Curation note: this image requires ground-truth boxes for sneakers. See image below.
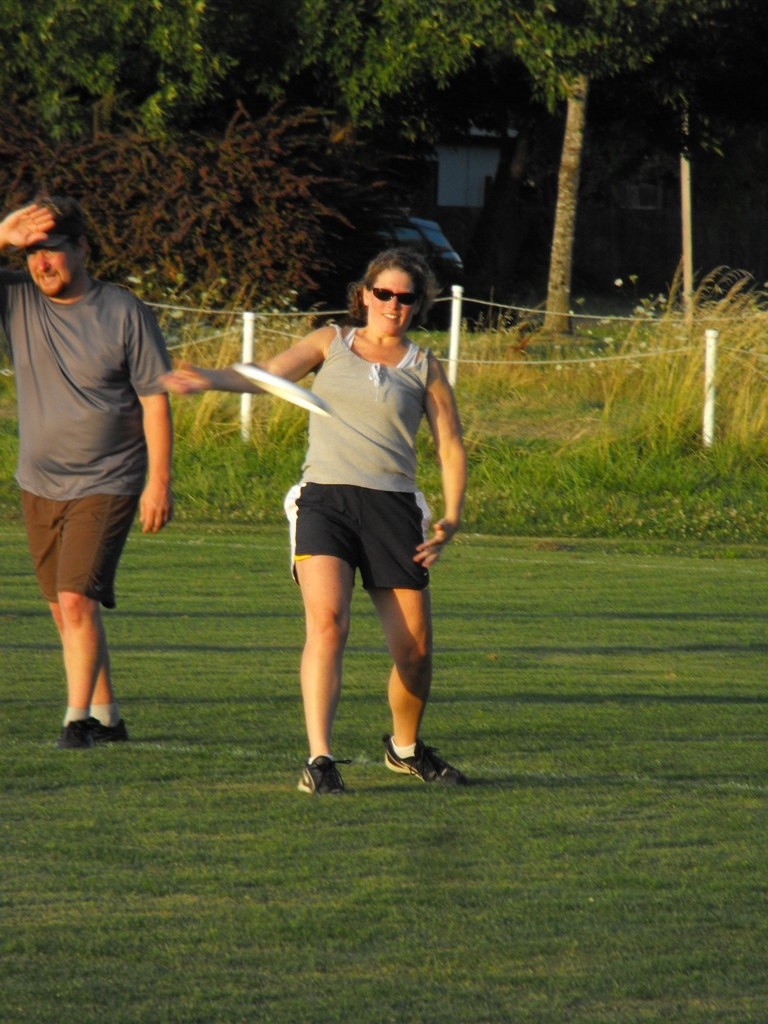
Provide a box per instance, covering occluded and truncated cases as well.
[381,733,467,786]
[85,714,129,745]
[58,717,89,749]
[295,755,352,795]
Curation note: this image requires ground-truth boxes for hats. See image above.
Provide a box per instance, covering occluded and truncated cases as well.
[11,232,68,252]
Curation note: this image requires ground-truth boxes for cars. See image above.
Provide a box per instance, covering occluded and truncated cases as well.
[308,212,464,330]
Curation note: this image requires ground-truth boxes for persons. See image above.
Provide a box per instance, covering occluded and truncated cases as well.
[1,197,176,747]
[156,246,468,790]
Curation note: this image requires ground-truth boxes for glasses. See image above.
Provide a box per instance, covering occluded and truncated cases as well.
[370,285,417,306]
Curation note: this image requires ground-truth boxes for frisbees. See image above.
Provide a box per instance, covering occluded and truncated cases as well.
[231,363,332,420]
